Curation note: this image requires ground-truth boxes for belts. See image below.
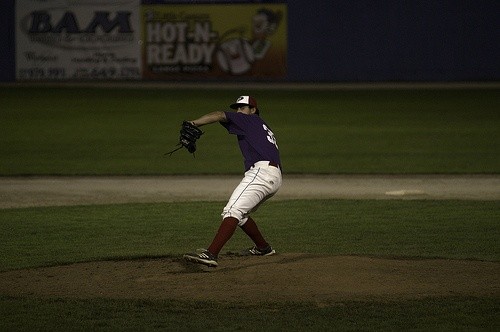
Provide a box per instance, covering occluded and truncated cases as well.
[253,161,279,168]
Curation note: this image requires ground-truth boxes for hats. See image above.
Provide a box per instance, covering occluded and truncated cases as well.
[229,95,257,109]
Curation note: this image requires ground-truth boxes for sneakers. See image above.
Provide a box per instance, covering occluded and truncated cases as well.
[183,248,218,268]
[242,244,275,255]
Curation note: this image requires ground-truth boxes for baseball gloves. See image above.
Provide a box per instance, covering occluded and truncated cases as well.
[178,120,204,155]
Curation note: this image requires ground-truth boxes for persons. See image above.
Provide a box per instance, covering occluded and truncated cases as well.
[181,96,283,266]
